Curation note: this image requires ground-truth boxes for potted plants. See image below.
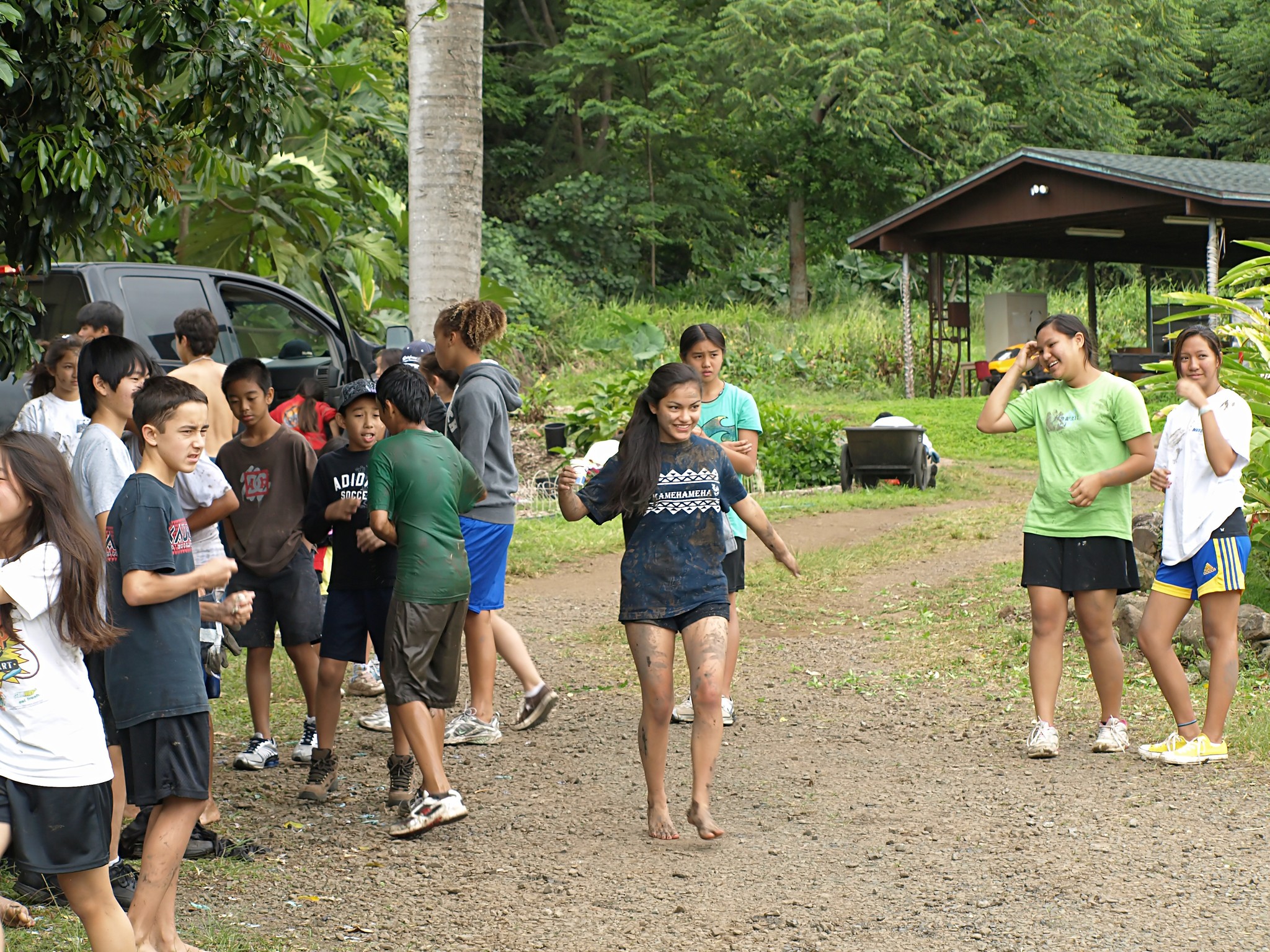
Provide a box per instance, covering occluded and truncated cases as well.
[534,472,558,500]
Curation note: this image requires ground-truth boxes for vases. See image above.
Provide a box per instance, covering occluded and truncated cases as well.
[545,423,567,455]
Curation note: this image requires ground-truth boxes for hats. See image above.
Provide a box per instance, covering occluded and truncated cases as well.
[278,339,315,359]
[337,379,377,412]
[400,340,435,369]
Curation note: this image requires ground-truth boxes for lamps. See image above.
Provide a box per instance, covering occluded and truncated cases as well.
[1030,184,1049,196]
[1162,215,1223,225]
[1065,227,1125,238]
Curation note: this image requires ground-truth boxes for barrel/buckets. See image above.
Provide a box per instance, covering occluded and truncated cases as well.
[535,478,559,499]
[544,423,566,455]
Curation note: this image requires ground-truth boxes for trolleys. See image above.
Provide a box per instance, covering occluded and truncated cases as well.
[840,425,929,491]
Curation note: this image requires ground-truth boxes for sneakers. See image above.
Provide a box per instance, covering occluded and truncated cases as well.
[109,857,140,912]
[1137,732,1189,760]
[349,654,385,697]
[671,687,694,721]
[134,834,213,859]
[233,732,279,769]
[1092,714,1130,753]
[1159,733,1228,764]
[358,705,392,733]
[1027,715,1059,757]
[443,698,502,744]
[389,790,468,839]
[291,716,318,762]
[12,869,70,907]
[509,683,560,731]
[398,786,423,817]
[721,695,736,724]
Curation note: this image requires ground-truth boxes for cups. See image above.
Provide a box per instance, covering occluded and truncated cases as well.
[570,459,589,486]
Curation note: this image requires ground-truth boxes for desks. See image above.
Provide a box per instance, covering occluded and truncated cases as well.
[958,362,976,397]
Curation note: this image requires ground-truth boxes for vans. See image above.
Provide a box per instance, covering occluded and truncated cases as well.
[0,259,414,470]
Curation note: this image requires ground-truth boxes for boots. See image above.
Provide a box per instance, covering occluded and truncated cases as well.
[387,754,415,806]
[297,747,338,802]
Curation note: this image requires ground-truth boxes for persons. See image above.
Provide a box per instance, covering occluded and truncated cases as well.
[658,323,763,724]
[872,411,939,489]
[977,315,1156,760]
[0,298,564,952]
[1136,324,1253,762]
[556,361,802,840]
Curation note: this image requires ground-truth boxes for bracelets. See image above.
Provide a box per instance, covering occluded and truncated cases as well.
[1198,404,1212,415]
